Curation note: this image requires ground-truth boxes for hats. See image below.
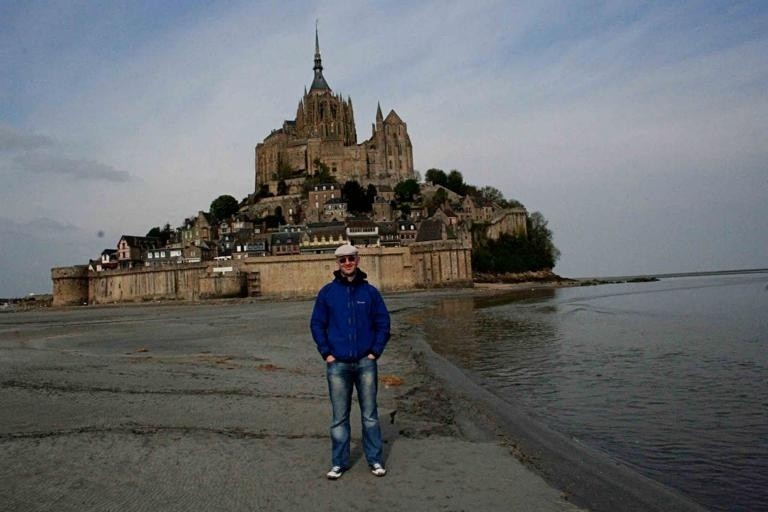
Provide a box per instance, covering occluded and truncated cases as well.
[333,244,359,256]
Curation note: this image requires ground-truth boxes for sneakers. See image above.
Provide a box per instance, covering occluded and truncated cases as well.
[325,465,346,479]
[368,461,387,476]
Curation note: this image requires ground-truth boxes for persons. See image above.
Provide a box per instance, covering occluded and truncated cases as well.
[310,244,390,480]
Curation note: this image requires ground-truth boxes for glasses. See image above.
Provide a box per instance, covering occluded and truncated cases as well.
[336,257,356,263]
